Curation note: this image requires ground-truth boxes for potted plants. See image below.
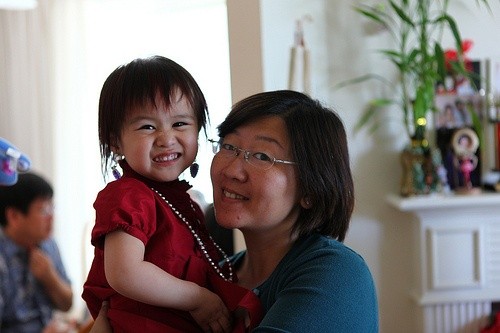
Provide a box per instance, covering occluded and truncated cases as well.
[339,0,495,196]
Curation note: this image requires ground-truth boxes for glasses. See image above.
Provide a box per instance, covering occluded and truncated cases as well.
[208,139,297,168]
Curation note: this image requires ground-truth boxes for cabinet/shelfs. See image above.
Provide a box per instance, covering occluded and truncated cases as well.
[387,191,500,333]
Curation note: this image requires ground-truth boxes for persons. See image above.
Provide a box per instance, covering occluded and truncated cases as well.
[0,171,80,333]
[80,55,225,333]
[82,87,382,333]
[430,66,485,189]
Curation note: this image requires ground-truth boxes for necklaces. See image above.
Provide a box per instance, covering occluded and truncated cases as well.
[144,184,233,285]
